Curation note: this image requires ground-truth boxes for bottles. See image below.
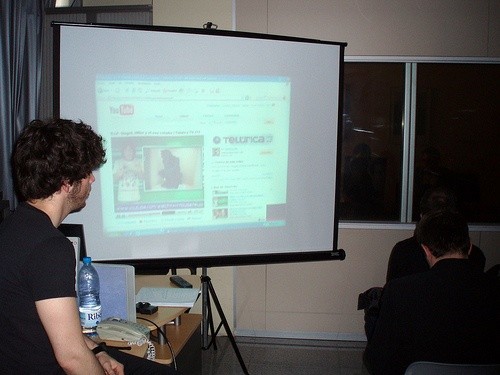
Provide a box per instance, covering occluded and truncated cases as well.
[77,257,102,339]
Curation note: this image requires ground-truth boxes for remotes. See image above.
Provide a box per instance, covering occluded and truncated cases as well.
[170,274,192,290]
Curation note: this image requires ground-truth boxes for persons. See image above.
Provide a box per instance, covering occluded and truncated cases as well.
[0,119,124,375]
[366,190,500,375]
[113,141,145,209]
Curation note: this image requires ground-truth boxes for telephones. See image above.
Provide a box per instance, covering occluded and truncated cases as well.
[96,318,148,342]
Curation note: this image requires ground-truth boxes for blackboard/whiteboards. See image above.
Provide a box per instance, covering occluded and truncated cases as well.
[338,55,500,230]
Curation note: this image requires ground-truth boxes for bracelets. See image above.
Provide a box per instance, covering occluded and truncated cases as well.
[91,346,105,356]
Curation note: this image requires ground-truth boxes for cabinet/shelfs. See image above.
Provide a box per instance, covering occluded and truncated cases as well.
[94,274,203,375]
